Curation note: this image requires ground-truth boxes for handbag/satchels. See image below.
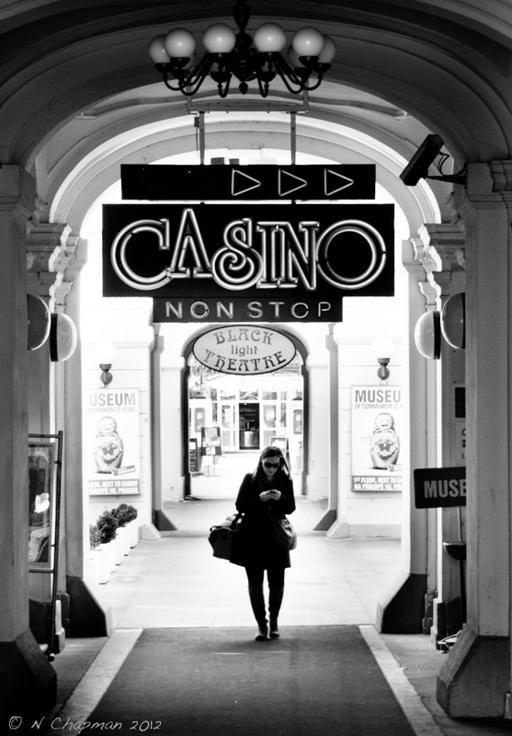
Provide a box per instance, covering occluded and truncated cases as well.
[279,516,298,550]
[207,514,242,560]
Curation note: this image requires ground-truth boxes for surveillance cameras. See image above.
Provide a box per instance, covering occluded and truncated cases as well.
[400,133,444,185]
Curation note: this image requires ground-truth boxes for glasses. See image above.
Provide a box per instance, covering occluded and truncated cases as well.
[264,462,280,468]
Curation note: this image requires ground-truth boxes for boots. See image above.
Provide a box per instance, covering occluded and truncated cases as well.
[254,616,280,642]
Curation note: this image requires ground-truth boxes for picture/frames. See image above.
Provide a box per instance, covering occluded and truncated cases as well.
[81,388,141,496]
[350,384,404,492]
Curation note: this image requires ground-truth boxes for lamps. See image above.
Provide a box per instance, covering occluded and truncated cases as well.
[51,310,78,363]
[100,363,112,386]
[27,293,50,352]
[149,0,336,96]
[414,310,441,360]
[377,358,390,380]
[441,293,466,349]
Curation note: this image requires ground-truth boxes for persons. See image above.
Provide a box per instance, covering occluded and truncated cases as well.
[235,444,298,642]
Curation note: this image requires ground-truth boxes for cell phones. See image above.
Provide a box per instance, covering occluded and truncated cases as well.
[268,490,276,494]
